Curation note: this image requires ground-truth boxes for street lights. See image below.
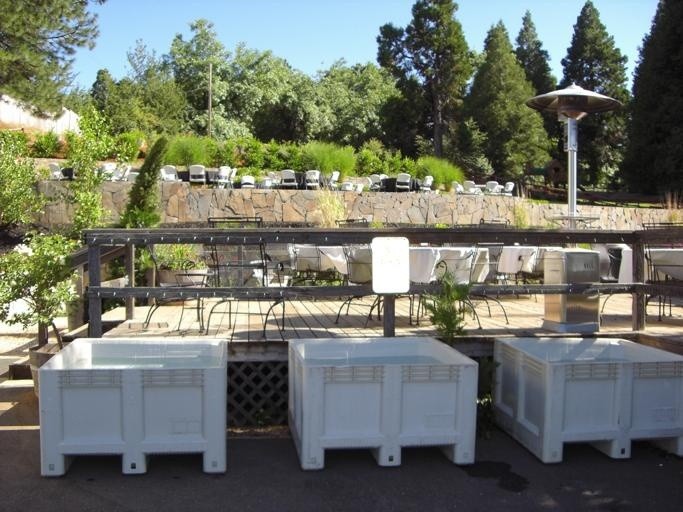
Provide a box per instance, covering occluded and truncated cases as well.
[525,80,622,248]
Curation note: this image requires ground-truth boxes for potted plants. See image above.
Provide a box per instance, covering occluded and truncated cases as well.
[0,231,80,398]
